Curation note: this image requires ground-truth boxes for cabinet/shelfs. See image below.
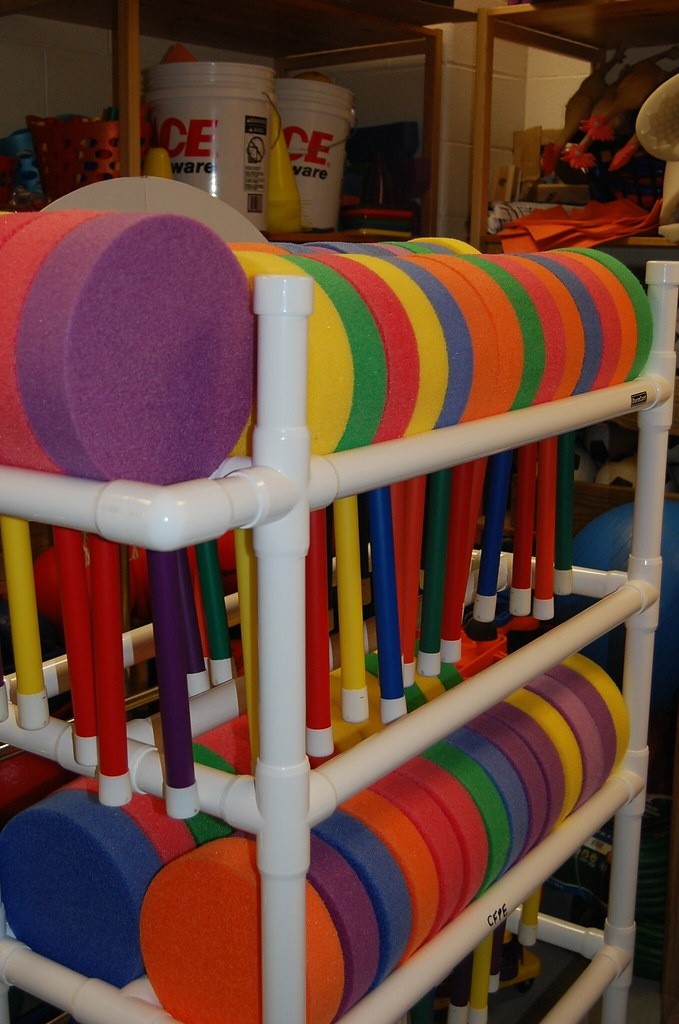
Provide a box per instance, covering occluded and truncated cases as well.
[1,0,679,1024]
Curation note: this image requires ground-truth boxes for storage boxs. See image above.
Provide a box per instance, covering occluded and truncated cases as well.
[513,127,590,205]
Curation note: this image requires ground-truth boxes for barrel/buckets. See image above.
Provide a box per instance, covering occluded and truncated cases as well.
[142,61,278,238]
[272,75,358,233]
